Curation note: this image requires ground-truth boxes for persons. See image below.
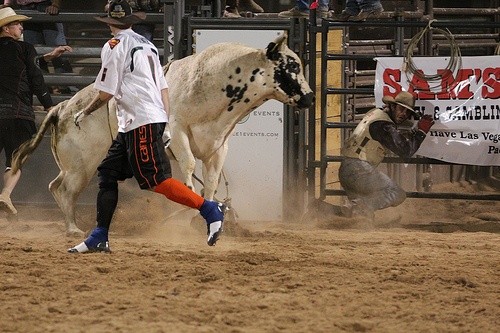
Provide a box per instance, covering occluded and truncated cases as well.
[0,7,56,225]
[277,0,385,21]
[68,0,228,254]
[223,0,265,18]
[306,91,436,227]
[0,0,80,95]
[108,0,165,44]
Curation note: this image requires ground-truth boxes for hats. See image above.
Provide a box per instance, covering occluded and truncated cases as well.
[0,6,32,28]
[96,0,147,26]
[382,91,420,117]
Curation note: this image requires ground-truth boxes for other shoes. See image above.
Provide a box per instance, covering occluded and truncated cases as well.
[349,7,385,22]
[332,13,351,21]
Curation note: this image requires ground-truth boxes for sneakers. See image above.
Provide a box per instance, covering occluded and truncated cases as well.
[278,7,309,20]
[316,10,331,19]
[223,6,241,18]
[67,227,109,253]
[200,200,227,246]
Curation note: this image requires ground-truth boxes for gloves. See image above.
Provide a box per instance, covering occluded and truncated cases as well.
[74,109,88,127]
[162,127,171,148]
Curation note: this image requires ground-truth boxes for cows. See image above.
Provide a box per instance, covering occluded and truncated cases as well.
[7,29,318,239]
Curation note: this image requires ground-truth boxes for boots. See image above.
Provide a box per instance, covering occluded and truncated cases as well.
[0,168,22,217]
